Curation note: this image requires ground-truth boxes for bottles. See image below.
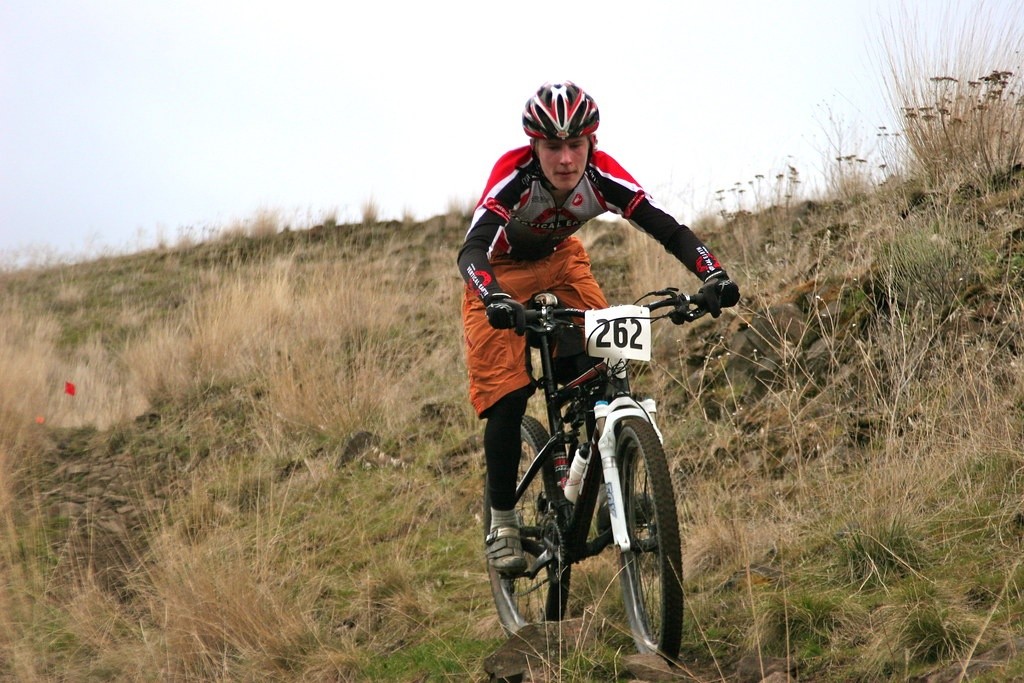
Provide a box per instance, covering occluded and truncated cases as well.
[564,441,591,504]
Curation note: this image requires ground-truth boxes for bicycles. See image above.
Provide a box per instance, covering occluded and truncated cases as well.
[481,287,729,666]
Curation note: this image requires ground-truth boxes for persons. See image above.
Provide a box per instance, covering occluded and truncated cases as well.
[466,80,741,571]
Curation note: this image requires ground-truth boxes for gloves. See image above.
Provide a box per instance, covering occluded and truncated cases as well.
[483,291,526,336]
[697,268,741,318]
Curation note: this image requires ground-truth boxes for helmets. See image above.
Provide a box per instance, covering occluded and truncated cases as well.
[522,81,599,139]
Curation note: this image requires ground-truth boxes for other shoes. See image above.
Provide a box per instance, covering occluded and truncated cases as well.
[486,524,528,575]
[598,498,661,553]
